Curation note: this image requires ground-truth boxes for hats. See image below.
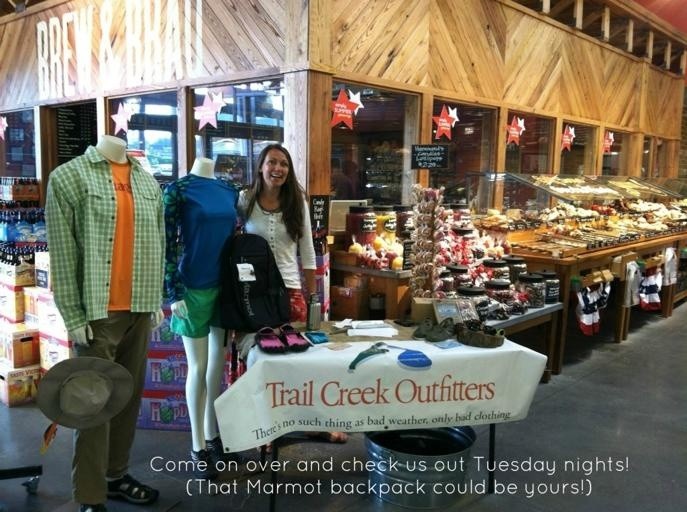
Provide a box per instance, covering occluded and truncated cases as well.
[35,356,135,431]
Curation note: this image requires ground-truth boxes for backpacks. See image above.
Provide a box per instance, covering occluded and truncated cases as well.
[220,233,292,334]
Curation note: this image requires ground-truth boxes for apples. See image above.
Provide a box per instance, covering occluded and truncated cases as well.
[385,252,399,267]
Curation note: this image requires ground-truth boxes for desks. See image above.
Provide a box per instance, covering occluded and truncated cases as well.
[329,256,416,324]
[479,299,563,383]
[206,311,549,511]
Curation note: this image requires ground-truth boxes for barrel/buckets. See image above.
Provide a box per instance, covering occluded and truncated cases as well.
[397,204,411,242]
[346,205,375,247]
[375,205,398,246]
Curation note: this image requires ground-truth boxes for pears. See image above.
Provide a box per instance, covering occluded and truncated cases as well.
[349,243,362,254]
[392,257,403,270]
[372,238,388,251]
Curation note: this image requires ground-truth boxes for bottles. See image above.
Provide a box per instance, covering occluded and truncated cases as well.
[306,293,322,330]
[346,203,560,324]
[0,175,39,208]
[0,207,47,267]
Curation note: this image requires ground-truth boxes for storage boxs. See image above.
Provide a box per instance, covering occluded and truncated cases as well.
[330,273,371,321]
[0,181,328,438]
[406,298,442,323]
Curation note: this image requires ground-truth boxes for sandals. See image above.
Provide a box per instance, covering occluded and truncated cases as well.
[312,431,349,445]
[104,473,159,504]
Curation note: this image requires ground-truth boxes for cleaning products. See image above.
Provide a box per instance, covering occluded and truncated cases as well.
[0,206,46,242]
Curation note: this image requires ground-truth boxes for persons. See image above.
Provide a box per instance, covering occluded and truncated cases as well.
[330,158,357,197]
[162,157,244,481]
[234,145,349,444]
[43,134,166,512]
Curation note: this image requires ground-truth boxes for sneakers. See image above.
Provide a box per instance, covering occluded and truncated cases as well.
[79,503,110,512]
[412,316,456,342]
[204,436,251,467]
[252,325,309,354]
[190,449,217,481]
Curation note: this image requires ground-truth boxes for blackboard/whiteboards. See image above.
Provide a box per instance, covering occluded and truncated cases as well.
[411,144,450,170]
[309,194,329,236]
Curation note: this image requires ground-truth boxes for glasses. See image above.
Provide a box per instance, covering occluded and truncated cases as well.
[468,321,497,336]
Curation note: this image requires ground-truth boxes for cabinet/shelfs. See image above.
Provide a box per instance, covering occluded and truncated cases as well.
[464,168,687,376]
